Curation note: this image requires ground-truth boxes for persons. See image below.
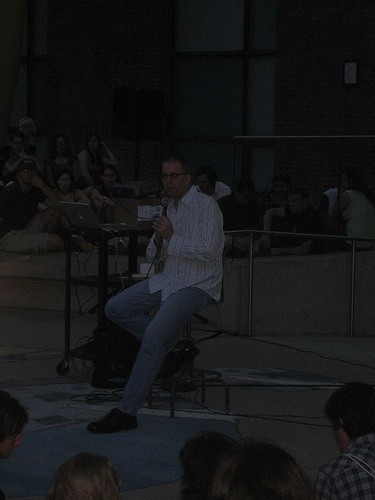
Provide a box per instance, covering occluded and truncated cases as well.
[0,390,29,500]
[46,453,122,500]
[178,431,240,500]
[309,382,375,500]
[0,116,375,259]
[206,443,314,500]
[88,155,225,433]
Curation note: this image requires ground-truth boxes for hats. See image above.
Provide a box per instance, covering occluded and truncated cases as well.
[17,159,39,171]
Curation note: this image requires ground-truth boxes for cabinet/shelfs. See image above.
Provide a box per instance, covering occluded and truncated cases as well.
[41,217,157,376]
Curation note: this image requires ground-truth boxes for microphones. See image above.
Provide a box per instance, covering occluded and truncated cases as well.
[161,198,169,216]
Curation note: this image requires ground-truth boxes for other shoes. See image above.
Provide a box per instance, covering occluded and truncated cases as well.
[85,409,137,432]
[164,342,200,376]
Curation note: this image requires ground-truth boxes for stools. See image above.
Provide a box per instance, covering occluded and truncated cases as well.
[147,275,231,417]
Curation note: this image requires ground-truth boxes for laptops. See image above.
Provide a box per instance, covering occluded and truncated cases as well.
[60,201,134,232]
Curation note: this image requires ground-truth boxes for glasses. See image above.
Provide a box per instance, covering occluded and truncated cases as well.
[161,172,182,178]
